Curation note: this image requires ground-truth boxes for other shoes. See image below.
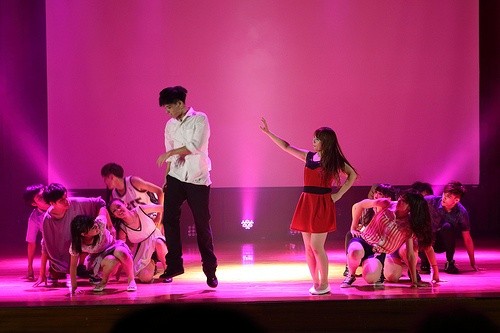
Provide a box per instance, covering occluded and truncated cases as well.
[158,267,184,281]
[93,283,103,291]
[444,259,460,274]
[421,259,430,274]
[339,274,356,287]
[343,266,349,276]
[309,284,331,296]
[374,279,385,289]
[206,271,218,289]
[127,281,137,292]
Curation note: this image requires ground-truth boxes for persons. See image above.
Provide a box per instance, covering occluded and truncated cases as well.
[23,162,169,296]
[259,116,358,295]
[158,85,219,288]
[340,181,486,288]
